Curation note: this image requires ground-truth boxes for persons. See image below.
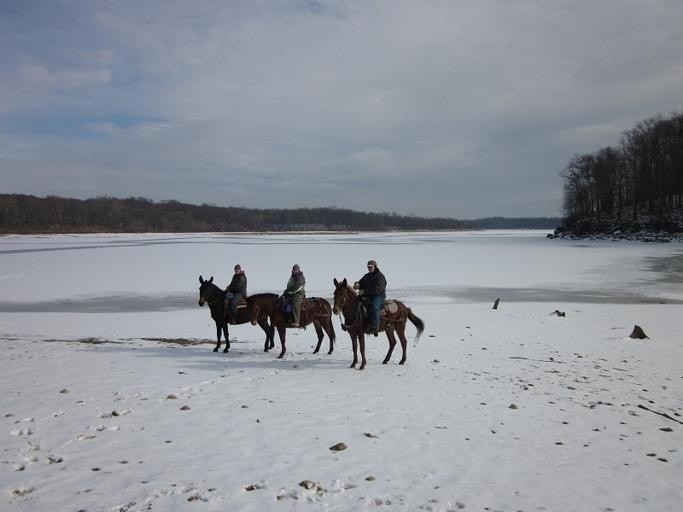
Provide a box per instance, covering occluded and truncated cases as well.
[224,264,247,324]
[283,264,306,327]
[352,259,387,337]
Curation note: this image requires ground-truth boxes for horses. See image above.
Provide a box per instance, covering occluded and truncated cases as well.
[198,275,279,353]
[332,278,424,370]
[246,293,336,358]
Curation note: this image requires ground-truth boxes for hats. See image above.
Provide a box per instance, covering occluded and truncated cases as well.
[234,265,240,271]
[368,261,376,267]
[292,265,299,270]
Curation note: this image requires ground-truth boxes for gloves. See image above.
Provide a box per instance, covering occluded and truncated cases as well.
[359,290,364,295]
[353,283,359,288]
